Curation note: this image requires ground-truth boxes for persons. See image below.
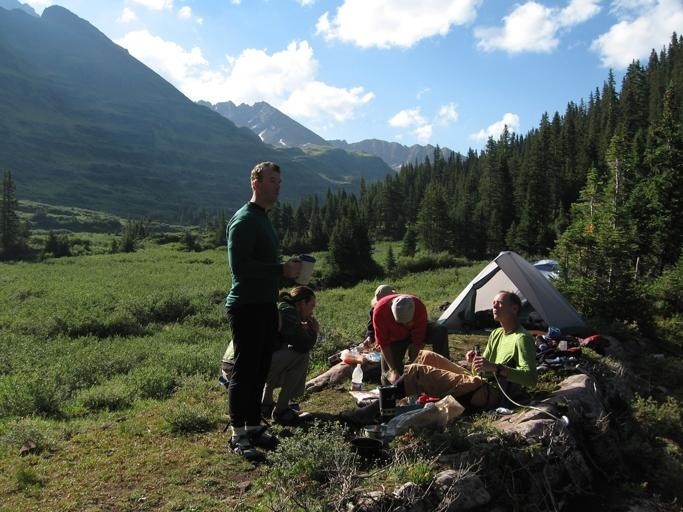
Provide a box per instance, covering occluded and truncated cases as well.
[220,285,321,425]
[341,290,539,425]
[372,294,451,387]
[306,284,398,392]
[225,162,301,463]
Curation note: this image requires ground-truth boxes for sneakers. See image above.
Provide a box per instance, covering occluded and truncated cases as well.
[342,409,377,425]
[360,400,379,411]
[229,402,313,461]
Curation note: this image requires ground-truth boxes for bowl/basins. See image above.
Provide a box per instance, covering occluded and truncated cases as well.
[348,438,384,451]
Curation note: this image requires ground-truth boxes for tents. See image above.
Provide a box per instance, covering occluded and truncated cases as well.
[533,259,576,280]
[437,250,588,335]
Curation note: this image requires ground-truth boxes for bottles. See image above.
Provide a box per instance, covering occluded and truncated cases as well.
[472,343,485,377]
[351,363,364,390]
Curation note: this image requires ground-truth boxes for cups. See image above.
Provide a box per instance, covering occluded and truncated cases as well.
[290,253,317,286]
[378,385,398,420]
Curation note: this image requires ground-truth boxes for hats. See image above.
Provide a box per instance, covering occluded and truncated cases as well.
[371,285,397,308]
[391,295,415,324]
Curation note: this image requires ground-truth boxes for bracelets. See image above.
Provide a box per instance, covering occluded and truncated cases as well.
[495,361,502,376]
